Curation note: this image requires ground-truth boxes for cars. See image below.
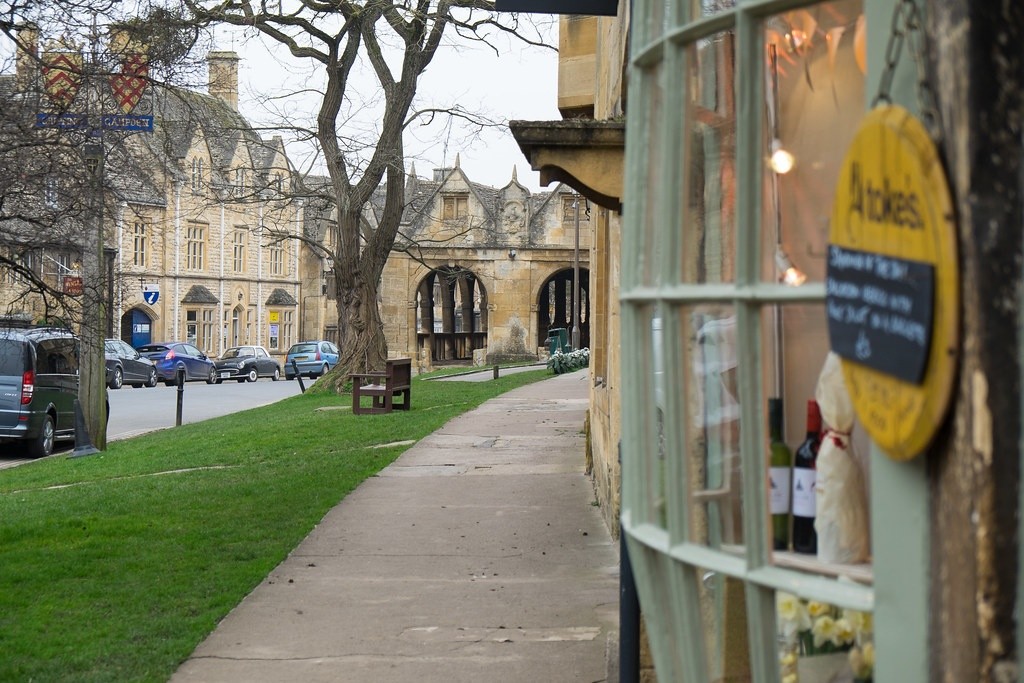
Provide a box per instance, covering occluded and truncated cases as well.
[215,346,281,384]
[104,338,159,390]
[136,342,218,386]
[284,340,339,381]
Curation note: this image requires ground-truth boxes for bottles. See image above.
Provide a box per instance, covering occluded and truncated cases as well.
[792,400,824,555]
[768,398,792,550]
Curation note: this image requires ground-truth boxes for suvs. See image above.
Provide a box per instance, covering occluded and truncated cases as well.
[0,326,111,458]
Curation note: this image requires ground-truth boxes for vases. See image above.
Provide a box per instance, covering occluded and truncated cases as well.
[798,652,845,683]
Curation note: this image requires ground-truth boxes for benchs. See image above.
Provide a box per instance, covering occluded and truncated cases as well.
[349,359,414,415]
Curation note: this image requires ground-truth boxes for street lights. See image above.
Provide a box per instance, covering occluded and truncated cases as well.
[256,190,268,345]
[172,174,183,344]
[219,185,228,354]
[570,188,581,352]
[117,192,129,341]
[294,196,303,340]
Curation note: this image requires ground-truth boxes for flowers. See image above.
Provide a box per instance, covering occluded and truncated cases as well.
[774,590,874,683]
